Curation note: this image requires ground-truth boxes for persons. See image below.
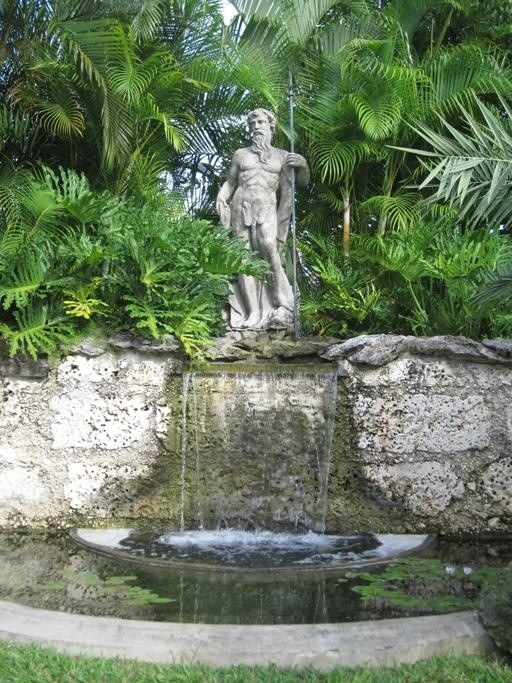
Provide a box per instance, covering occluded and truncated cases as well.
[212,109,315,337]
